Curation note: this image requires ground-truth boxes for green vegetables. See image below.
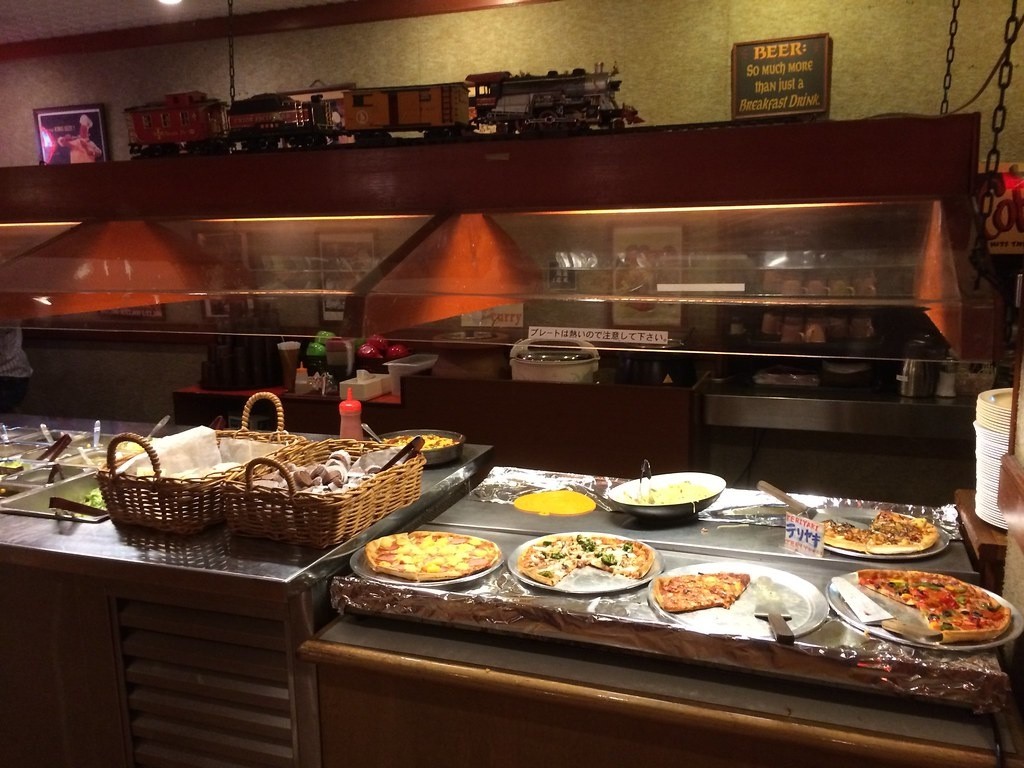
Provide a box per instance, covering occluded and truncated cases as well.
[82,487,107,510]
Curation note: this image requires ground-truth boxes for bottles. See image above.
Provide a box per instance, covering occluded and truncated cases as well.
[295,363,311,395]
[901,339,957,398]
[340,390,363,443]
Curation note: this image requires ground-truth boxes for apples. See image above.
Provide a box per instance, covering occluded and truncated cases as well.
[306,330,338,355]
[354,334,410,359]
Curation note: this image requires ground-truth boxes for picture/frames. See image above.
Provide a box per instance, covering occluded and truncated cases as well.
[32,103,109,165]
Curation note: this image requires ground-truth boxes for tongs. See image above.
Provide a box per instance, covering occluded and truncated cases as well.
[210,415,226,431]
[50,495,107,517]
[38,434,72,460]
[377,437,425,474]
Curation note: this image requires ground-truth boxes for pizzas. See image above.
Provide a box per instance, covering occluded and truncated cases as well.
[380,434,460,450]
[365,530,501,581]
[818,512,939,554]
[855,569,1010,642]
[653,572,751,613]
[517,533,655,586]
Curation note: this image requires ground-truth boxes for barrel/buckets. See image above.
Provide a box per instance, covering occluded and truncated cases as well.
[616,337,695,390]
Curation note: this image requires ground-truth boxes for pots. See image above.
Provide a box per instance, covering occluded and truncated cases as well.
[510,336,600,384]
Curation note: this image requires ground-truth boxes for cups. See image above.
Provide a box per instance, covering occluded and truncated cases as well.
[765,270,878,297]
[762,308,875,342]
[277,341,300,388]
[326,336,355,378]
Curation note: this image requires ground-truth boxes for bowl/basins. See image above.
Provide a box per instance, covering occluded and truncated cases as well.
[374,430,466,465]
[609,472,727,524]
[360,359,396,372]
[302,355,326,372]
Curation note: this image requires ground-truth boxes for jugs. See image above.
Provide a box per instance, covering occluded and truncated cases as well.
[727,316,747,352]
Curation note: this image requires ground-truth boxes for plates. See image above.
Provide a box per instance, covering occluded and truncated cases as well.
[350,546,502,586]
[648,561,831,636]
[827,570,1023,653]
[504,531,665,594]
[972,388,1013,530]
[792,508,951,558]
